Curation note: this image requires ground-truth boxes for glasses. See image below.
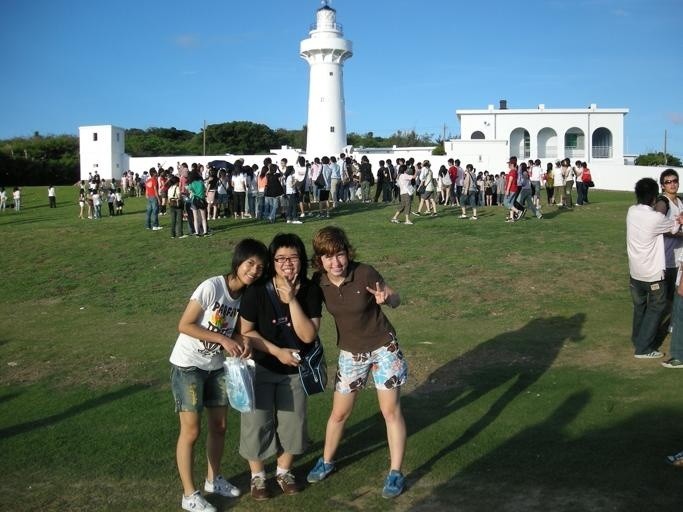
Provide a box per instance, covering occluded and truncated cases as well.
[664,179,679,185]
[274,255,300,263]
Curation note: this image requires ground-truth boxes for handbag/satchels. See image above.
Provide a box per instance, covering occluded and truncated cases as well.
[193,195,208,209]
[416,177,433,195]
[581,177,594,187]
[343,161,350,185]
[169,185,183,209]
[295,166,309,195]
[314,165,326,188]
[219,179,233,201]
[406,184,415,196]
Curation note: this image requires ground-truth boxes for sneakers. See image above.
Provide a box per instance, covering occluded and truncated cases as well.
[536,210,543,220]
[205,475,242,498]
[411,211,421,217]
[515,210,523,220]
[391,217,400,224]
[275,470,300,496]
[634,351,665,359]
[429,206,433,210]
[291,220,303,225]
[179,235,189,239]
[666,451,683,467]
[523,207,527,220]
[263,278,328,396]
[662,358,683,369]
[146,227,151,230]
[250,474,271,503]
[307,213,314,217]
[287,220,292,223]
[469,217,478,221]
[195,234,200,238]
[170,236,176,239]
[202,232,212,237]
[299,214,305,218]
[153,226,163,231]
[505,217,514,222]
[326,211,331,218]
[423,211,432,215]
[307,457,338,483]
[382,470,406,499]
[431,213,438,217]
[457,214,467,219]
[316,214,325,218]
[181,490,217,512]
[404,221,413,225]
[268,216,274,224]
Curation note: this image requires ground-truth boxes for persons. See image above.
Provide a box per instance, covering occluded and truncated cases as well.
[294,156,314,217]
[561,160,567,178]
[345,156,374,204]
[231,167,247,220]
[409,158,415,185]
[168,239,268,512]
[279,158,287,218]
[405,161,413,175]
[107,191,116,216]
[563,158,575,209]
[314,156,332,218]
[80,181,86,191]
[89,172,93,181]
[79,189,85,219]
[92,190,102,219]
[145,168,163,230]
[507,157,526,219]
[92,182,98,193]
[436,177,441,204]
[662,213,683,369]
[572,160,584,206]
[390,166,418,225]
[504,160,522,222]
[181,163,189,176]
[440,165,452,205]
[551,161,563,206]
[413,161,438,217]
[204,170,218,219]
[520,164,543,218]
[13,186,21,211]
[85,192,95,218]
[373,160,386,202]
[186,174,208,235]
[305,160,314,168]
[457,164,477,220]
[236,232,322,500]
[101,179,107,191]
[246,167,257,218]
[264,164,283,223]
[476,171,505,206]
[216,170,230,219]
[308,227,407,500]
[527,160,533,178]
[330,157,341,207]
[455,159,464,207]
[383,159,394,203]
[141,172,147,183]
[394,159,400,202]
[120,170,134,193]
[169,167,173,175]
[424,198,434,214]
[447,159,457,206]
[158,168,164,196]
[192,161,211,178]
[167,177,188,239]
[310,158,321,203]
[264,158,272,167]
[94,171,100,184]
[625,178,683,359]
[133,173,141,197]
[659,170,683,332]
[284,166,304,224]
[48,184,56,208]
[115,188,124,215]
[111,178,117,193]
[397,159,405,173]
[257,166,269,220]
[252,164,258,172]
[0,187,7,211]
[160,170,170,215]
[581,162,594,202]
[655,197,669,336]
[543,163,554,204]
[88,179,93,191]
[532,159,542,209]
[337,153,346,202]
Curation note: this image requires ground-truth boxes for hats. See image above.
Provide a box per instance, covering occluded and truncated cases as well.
[506,159,517,165]
